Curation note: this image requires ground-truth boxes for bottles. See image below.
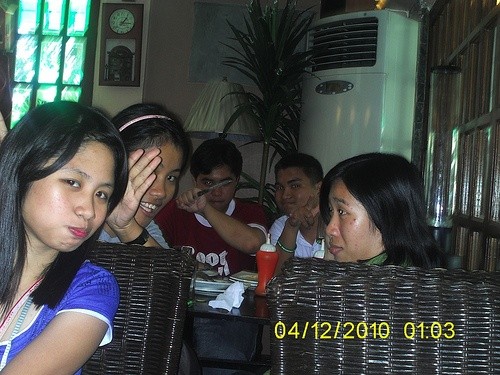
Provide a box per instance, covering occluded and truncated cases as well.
[257,233,279,296]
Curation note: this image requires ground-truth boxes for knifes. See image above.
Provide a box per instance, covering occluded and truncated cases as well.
[193,180,231,198]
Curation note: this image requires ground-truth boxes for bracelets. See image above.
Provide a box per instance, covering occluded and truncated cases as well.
[125,228,149,245]
[277,238,297,253]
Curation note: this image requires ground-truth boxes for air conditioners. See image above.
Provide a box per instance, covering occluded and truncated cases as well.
[293,13,421,176]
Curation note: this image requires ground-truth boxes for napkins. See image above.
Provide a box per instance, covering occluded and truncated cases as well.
[209,280,246,312]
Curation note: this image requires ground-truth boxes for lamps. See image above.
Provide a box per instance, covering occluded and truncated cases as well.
[184,75,263,144]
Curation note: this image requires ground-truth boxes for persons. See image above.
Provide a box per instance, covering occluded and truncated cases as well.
[268,153,324,277]
[0,100,129,375]
[96,102,189,248]
[319,153,441,268]
[152,139,269,280]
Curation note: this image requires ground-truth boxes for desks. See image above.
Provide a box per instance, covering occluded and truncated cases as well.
[183,270,271,375]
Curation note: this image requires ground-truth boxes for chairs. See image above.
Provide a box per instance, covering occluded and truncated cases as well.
[80,243,192,375]
[262,251,500,375]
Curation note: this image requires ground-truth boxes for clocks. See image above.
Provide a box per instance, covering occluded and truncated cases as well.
[97,2,145,89]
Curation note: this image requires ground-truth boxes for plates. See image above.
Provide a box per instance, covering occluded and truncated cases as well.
[190,287,225,297]
[195,278,231,289]
[229,276,258,289]
